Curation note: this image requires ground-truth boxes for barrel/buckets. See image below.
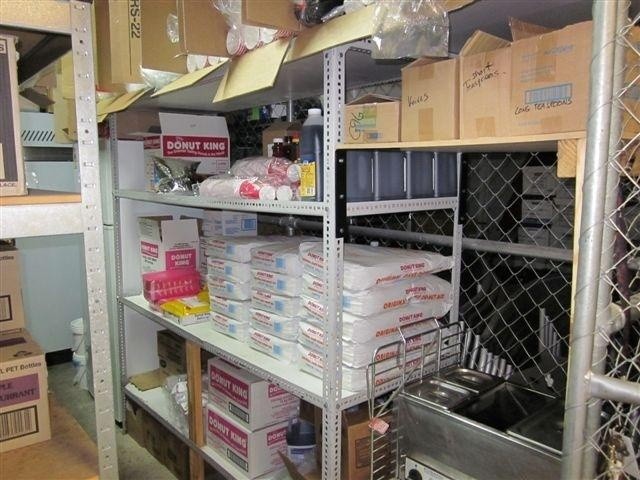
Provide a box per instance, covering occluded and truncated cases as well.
[69,317,86,353]
[72,353,88,390]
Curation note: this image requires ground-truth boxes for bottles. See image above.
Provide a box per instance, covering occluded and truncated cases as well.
[271,133,300,163]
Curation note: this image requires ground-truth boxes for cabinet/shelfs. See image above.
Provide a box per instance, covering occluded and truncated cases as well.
[0,1,640,480]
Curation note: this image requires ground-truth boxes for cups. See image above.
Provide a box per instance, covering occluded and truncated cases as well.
[186,54,230,71]
[198,156,302,202]
[226,22,297,56]
